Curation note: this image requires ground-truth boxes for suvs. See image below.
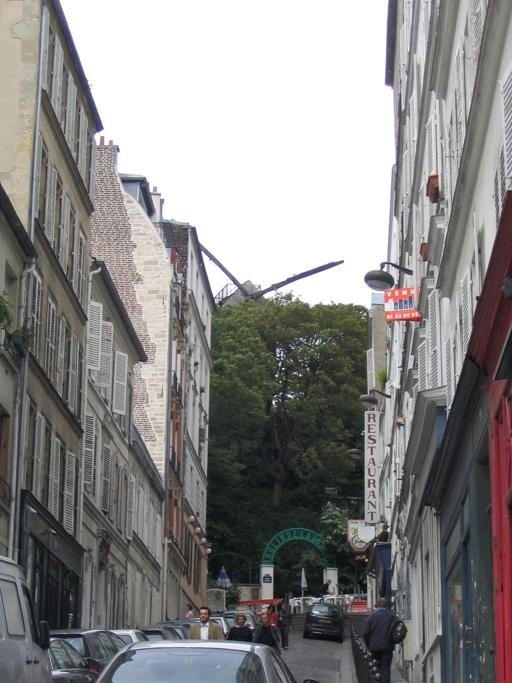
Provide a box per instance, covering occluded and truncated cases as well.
[0,555,55,683]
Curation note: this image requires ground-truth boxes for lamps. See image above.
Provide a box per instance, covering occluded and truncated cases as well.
[363,261,414,292]
[187,514,211,556]
[358,388,391,408]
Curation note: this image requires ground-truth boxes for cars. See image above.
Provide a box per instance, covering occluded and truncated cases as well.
[94,638,319,683]
[47,637,95,683]
[111,629,151,644]
[48,629,128,675]
[132,609,260,643]
[302,599,346,642]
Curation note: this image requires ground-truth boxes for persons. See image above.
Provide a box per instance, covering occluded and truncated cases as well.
[254,610,282,656]
[277,602,293,649]
[184,602,193,618]
[361,597,400,683]
[317,579,331,594]
[187,605,225,640]
[266,603,278,627]
[227,612,253,642]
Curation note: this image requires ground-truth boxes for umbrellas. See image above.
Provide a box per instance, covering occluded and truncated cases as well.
[300,567,308,596]
[213,565,233,609]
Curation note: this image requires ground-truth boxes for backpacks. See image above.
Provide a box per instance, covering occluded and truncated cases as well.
[389,613,407,644]
